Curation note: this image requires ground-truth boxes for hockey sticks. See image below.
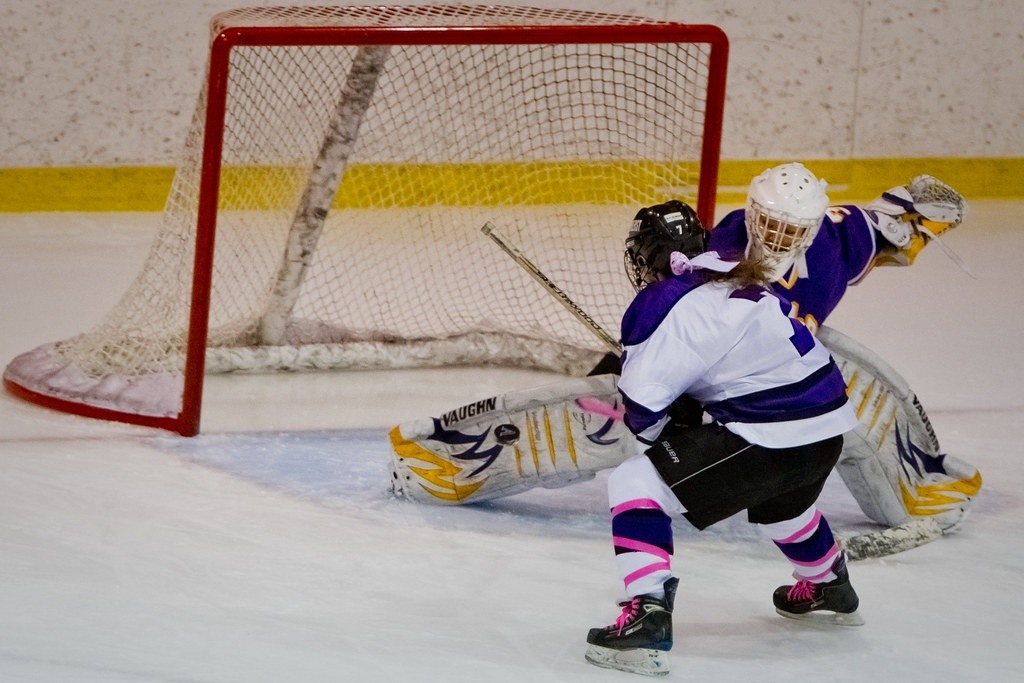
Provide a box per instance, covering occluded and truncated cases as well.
[478,217,946,567]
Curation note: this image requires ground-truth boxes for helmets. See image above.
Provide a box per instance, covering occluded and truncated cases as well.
[625,199,708,276]
[745,162,831,285]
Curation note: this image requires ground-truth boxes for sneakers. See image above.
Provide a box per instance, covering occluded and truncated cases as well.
[584,575,680,677]
[774,547,865,630]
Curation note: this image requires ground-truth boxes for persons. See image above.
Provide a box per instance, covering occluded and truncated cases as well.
[388,157,981,678]
